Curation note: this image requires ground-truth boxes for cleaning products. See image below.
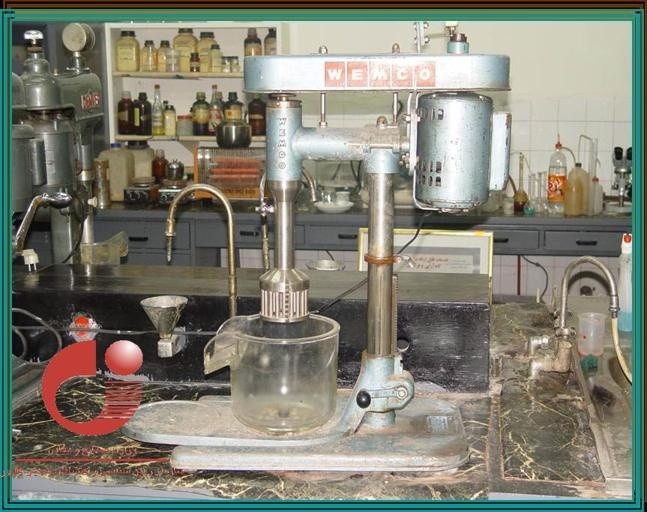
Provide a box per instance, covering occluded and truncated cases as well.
[616,232,633,331]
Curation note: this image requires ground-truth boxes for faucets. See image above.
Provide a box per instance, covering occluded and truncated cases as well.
[163,182,239,318]
[257,166,318,204]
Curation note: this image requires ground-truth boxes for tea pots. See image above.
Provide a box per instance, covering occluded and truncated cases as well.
[211,92,251,147]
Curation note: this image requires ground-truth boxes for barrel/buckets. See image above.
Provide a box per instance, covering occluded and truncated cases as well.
[568,163,589,215]
[123,139,154,180]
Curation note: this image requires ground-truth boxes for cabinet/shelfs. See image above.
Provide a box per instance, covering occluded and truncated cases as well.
[13,209,632,316]
[105,20,290,175]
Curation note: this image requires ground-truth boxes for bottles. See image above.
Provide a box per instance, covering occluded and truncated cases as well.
[186,173,195,201]
[514,139,605,214]
[113,31,278,136]
[618,233,633,332]
[447,34,468,54]
[153,149,166,176]
[127,140,155,189]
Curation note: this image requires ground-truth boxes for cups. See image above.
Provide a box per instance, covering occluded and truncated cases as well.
[227,314,337,433]
[79,242,121,266]
[168,160,183,181]
